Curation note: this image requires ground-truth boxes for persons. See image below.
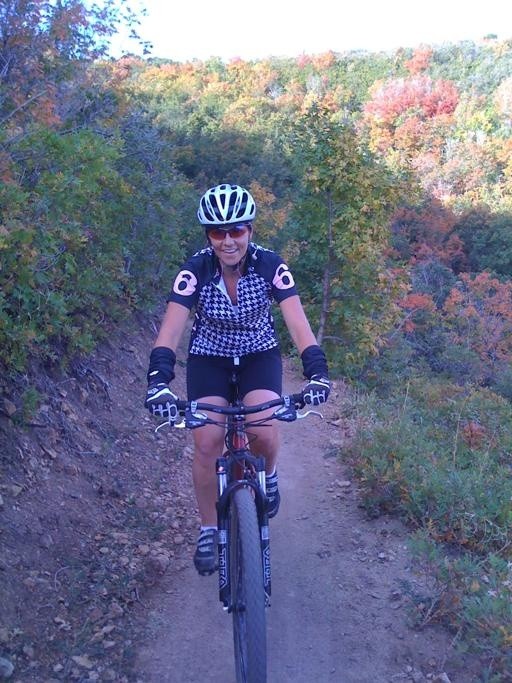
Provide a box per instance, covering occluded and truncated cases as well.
[145,184,331,572]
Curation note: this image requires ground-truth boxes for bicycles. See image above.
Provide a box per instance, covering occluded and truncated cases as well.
[155,390,323,683]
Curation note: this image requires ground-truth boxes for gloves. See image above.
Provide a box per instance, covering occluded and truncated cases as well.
[144,382,180,427]
[302,374,331,406]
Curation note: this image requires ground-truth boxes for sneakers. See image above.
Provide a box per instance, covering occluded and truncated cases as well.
[265,470,281,518]
[193,528,218,572]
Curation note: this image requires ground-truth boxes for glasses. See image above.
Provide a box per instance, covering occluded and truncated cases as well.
[207,227,249,240]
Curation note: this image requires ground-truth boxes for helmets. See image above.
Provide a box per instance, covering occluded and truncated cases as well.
[196,183,257,226]
